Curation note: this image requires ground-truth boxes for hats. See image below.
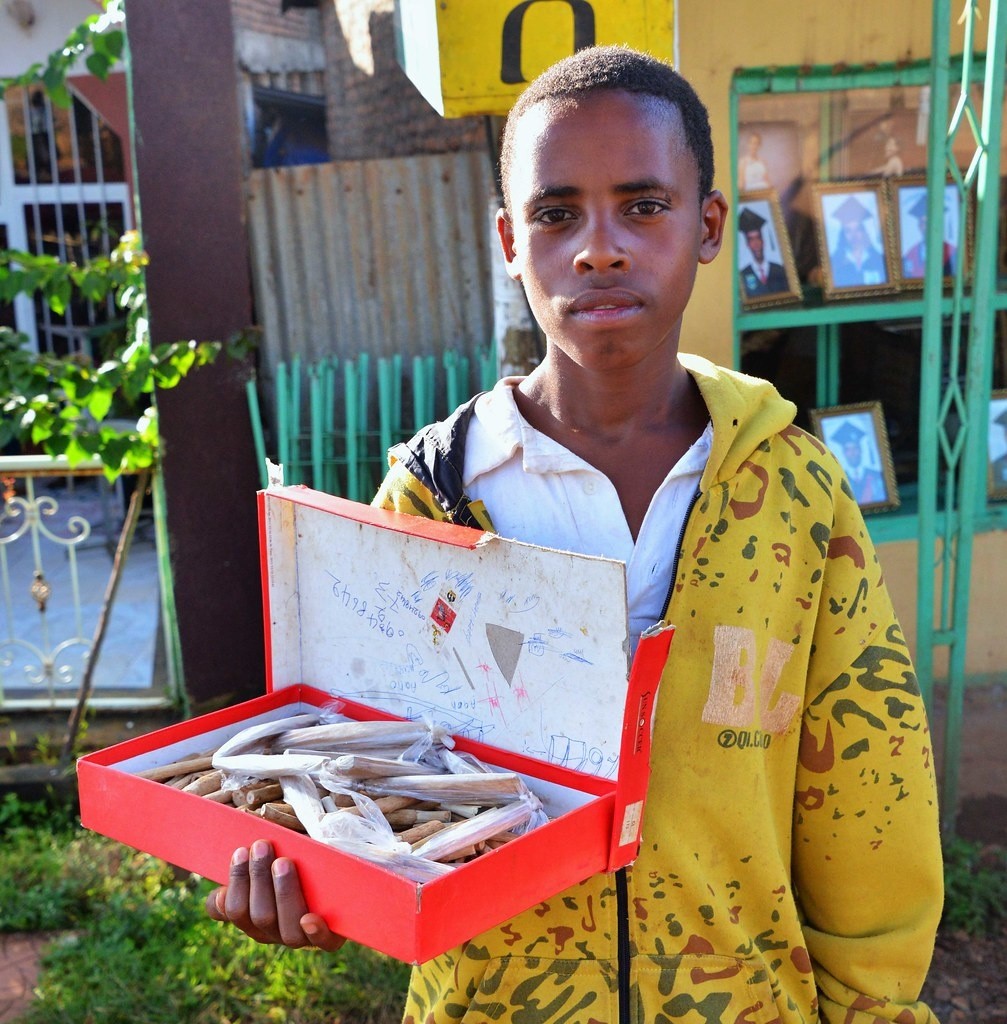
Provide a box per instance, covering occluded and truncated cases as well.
[907,193,953,240]
[832,420,875,465]
[739,209,776,252]
[832,196,880,242]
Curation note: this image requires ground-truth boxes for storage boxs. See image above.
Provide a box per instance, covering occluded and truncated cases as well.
[74,482,678,966]
[812,400,903,517]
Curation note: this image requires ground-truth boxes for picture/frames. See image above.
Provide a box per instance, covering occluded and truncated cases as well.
[807,179,900,300]
[738,189,805,312]
[890,173,973,290]
[965,390,1007,501]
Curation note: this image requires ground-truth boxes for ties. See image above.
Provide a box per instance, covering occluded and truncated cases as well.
[758,265,767,284]
[854,467,861,481]
[852,244,862,267]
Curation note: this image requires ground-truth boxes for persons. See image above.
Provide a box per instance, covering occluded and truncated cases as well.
[207,47,944,1024]
[902,195,957,279]
[739,208,790,297]
[832,423,888,505]
[866,136,904,178]
[738,133,771,191]
[830,198,885,287]
[993,409,1007,486]
